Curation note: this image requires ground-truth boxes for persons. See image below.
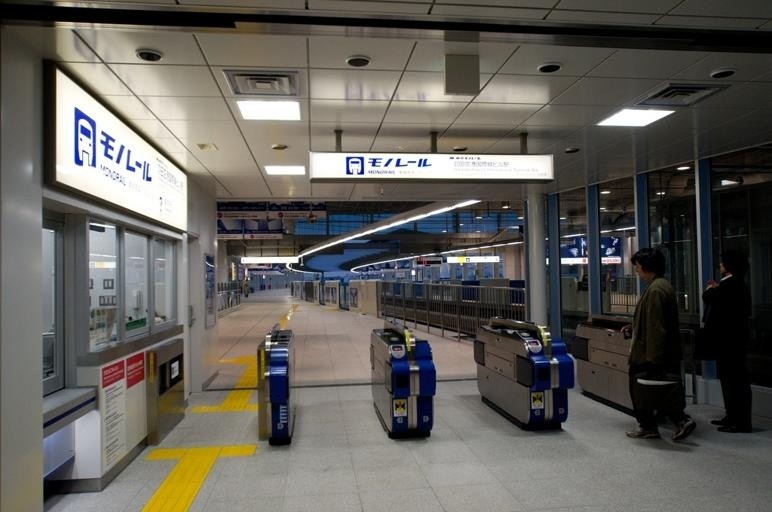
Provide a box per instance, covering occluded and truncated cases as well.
[699,252,755,433]
[621,247,697,443]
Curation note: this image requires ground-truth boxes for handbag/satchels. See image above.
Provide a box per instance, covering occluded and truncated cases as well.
[634,368,682,415]
[694,329,716,361]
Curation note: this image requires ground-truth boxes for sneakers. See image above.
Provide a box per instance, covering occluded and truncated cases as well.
[626,428,660,440]
[671,417,696,440]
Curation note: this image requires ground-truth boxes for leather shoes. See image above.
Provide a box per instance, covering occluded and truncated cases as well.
[712,417,753,432]
